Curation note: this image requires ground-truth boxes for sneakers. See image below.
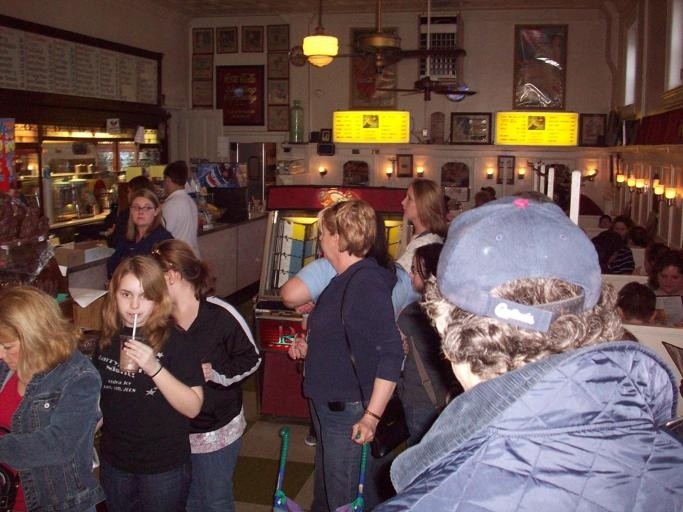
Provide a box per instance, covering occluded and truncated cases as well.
[305,432,318,446]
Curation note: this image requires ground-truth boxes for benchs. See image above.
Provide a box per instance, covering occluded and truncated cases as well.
[576,214,682,421]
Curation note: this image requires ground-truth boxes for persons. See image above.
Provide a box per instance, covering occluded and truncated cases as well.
[107,160,201,281]
[0,285,106,512]
[370,195,683,512]
[475,187,496,207]
[280,181,465,512]
[591,215,683,371]
[154,241,265,512]
[94,255,207,512]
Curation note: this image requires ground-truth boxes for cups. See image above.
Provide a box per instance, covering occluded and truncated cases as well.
[119,335,144,374]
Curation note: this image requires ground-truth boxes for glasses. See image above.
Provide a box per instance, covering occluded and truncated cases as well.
[128,206,155,211]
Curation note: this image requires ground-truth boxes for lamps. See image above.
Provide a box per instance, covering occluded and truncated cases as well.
[517,167,527,179]
[386,167,393,178]
[302,0,338,68]
[318,166,327,176]
[486,168,494,179]
[613,173,678,207]
[416,165,424,177]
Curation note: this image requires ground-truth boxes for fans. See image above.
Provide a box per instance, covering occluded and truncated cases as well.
[335,1,468,76]
[377,1,478,101]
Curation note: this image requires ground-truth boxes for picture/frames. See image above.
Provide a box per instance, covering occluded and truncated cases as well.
[350,26,399,109]
[580,113,608,146]
[319,128,332,143]
[495,154,515,186]
[512,23,568,110]
[449,111,492,145]
[192,23,291,133]
[396,153,413,177]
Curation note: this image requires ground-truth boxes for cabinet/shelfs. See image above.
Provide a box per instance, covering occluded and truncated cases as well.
[248,293,316,426]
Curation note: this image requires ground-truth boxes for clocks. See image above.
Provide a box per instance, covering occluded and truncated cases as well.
[289,45,307,67]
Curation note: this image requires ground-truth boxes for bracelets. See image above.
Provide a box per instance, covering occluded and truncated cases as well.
[150,365,163,378]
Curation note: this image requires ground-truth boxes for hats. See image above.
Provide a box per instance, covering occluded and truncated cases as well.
[435,195,603,333]
[481,186,495,195]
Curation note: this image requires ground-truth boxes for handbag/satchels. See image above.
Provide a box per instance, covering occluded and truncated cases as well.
[0,466,20,508]
[364,392,411,459]
